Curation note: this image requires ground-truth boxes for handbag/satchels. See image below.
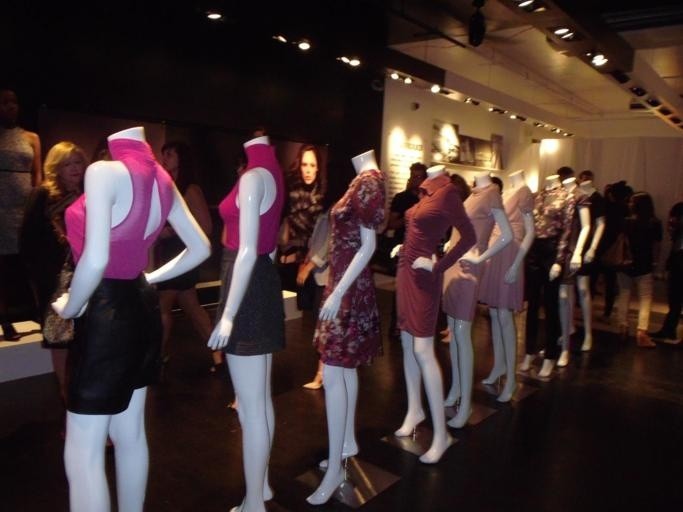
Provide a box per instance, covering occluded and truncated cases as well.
[614,233,634,266]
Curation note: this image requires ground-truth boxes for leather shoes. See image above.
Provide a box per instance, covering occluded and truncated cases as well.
[302,382,323,390]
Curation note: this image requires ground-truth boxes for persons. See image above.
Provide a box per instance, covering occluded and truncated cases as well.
[0,88,41,255]
[152,136,223,380]
[391,164,606,463]
[206,136,286,512]
[306,149,386,505]
[276,139,327,290]
[52,125,213,511]
[606,165,682,346]
[18,140,89,322]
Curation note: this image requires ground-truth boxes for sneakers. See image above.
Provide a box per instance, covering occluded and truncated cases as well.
[636,331,678,347]
